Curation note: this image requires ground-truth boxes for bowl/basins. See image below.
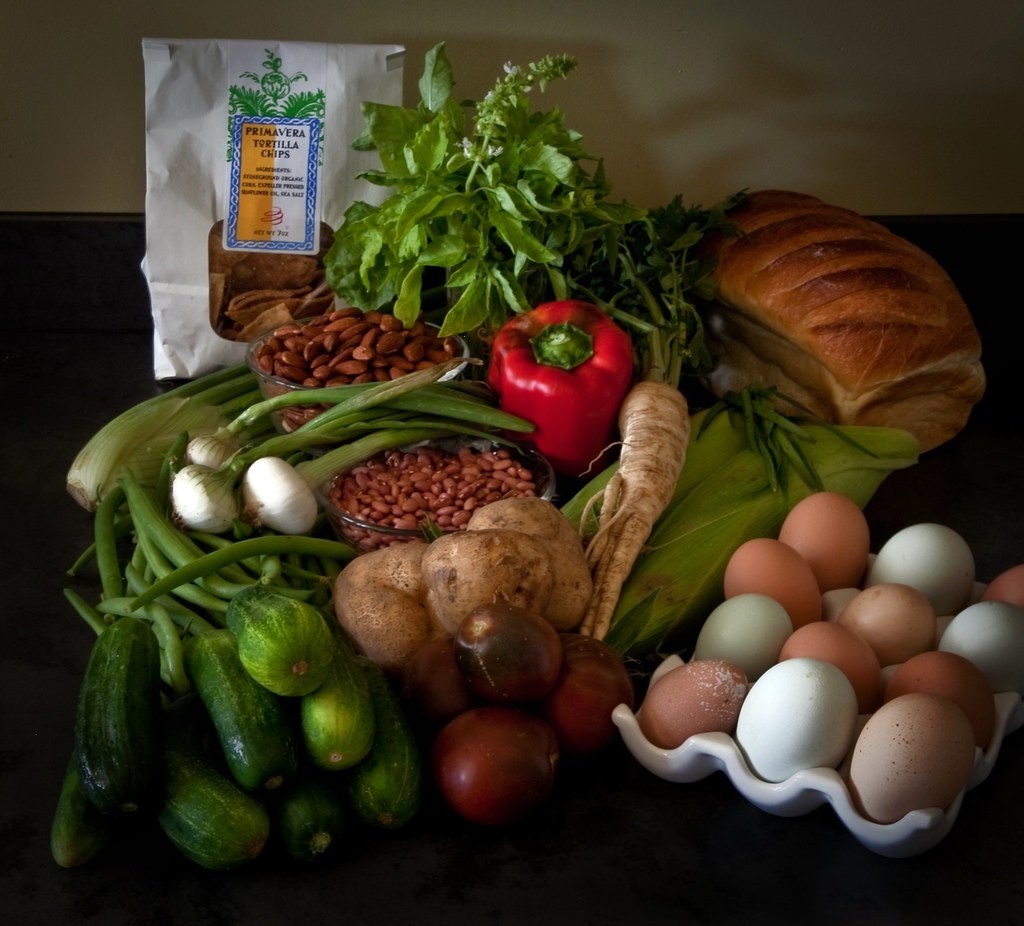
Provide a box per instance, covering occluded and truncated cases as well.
[245,315,470,434]
[315,436,558,553]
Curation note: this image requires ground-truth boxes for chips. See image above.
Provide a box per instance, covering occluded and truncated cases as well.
[209,221,334,341]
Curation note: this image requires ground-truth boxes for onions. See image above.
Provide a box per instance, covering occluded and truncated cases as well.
[241,456,318,535]
[186,435,244,468]
[171,462,238,533]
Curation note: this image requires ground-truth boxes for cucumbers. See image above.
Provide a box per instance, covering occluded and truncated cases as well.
[50,586,419,869]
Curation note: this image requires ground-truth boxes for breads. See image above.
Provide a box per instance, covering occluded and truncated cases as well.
[700,191,986,454]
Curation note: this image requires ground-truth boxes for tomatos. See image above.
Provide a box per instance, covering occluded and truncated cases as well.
[402,604,634,821]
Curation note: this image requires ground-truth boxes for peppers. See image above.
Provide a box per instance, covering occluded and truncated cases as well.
[490,300,631,471]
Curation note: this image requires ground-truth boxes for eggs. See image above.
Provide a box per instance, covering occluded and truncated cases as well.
[642,493,1024,824]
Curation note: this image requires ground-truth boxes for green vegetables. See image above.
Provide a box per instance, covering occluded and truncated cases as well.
[325,40,653,343]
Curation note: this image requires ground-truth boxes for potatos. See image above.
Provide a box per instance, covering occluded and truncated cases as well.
[334,497,592,669]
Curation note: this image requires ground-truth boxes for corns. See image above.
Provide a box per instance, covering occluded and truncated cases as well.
[557,405,920,655]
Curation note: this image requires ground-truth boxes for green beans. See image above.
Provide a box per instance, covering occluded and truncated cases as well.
[66,431,355,709]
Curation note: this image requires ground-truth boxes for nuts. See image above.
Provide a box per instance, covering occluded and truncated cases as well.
[257,307,459,432]
